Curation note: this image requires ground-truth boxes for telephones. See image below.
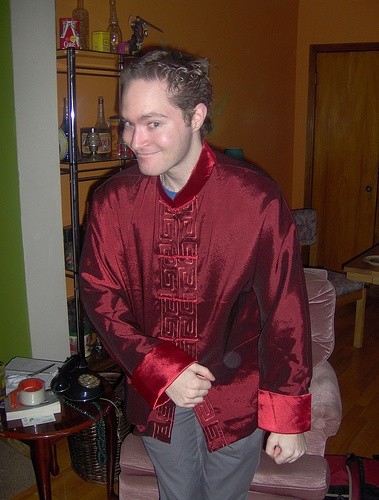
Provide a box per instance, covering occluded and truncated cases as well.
[50,354,107,403]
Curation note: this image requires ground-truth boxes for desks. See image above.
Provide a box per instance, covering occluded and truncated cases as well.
[342,245,379,349]
[0,387,101,500]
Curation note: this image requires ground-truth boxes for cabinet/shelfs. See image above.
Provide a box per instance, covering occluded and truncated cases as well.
[56,47,139,374]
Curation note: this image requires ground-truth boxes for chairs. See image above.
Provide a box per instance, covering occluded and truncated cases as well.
[292,209,365,307]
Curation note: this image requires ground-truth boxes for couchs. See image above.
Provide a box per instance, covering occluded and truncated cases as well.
[118,269,342,500]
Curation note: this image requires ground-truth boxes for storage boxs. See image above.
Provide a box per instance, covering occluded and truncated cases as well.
[59,18,80,49]
[118,42,129,54]
[93,32,110,52]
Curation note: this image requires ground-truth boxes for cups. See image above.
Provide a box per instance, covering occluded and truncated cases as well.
[0,361,7,409]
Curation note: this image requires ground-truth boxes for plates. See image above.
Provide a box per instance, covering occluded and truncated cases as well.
[362,256,379,266]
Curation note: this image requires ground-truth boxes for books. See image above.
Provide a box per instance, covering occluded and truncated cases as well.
[4,391,61,421]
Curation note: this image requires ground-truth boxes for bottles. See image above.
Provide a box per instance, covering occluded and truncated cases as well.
[107,0,122,54]
[117,136,132,158]
[72,0,89,51]
[95,95,112,159]
[58,97,70,162]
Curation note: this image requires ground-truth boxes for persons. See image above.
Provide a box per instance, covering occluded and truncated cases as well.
[78,49,313,500]
[128,15,148,46]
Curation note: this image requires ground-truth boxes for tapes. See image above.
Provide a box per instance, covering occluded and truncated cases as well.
[18,378,46,406]
